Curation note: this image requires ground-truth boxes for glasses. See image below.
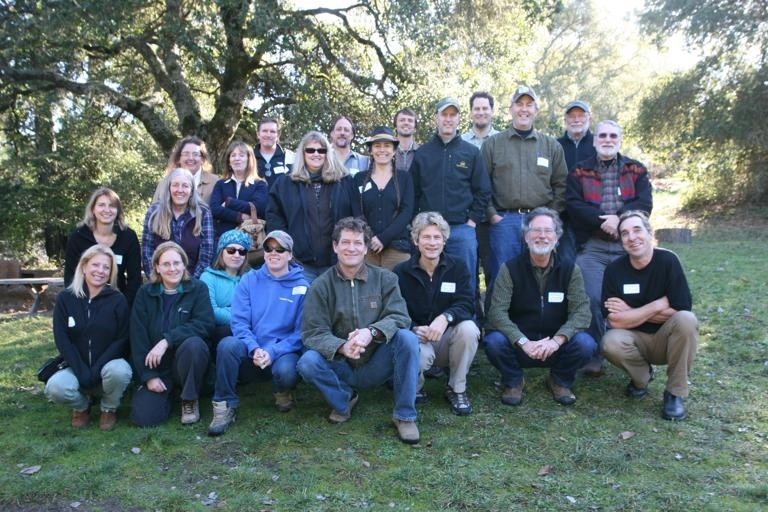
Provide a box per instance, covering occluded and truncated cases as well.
[598,132,617,138]
[264,163,272,177]
[263,243,286,254]
[304,147,328,154]
[180,151,202,158]
[225,247,248,256]
[527,227,557,234]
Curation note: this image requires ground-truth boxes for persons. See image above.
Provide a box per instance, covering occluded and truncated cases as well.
[395,85,597,421]
[145,167,213,280]
[43,242,134,431]
[130,241,215,425]
[600,212,698,421]
[64,187,144,312]
[171,113,421,441]
[565,120,653,374]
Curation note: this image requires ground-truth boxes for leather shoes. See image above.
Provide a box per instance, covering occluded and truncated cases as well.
[625,365,656,397]
[663,388,686,421]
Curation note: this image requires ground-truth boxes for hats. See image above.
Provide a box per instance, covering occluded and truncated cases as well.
[217,230,250,252]
[512,86,537,103]
[262,229,293,250]
[436,97,460,113]
[565,101,588,112]
[362,126,400,144]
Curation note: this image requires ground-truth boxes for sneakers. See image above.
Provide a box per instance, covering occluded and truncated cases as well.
[273,391,293,413]
[502,376,525,405]
[180,399,200,425]
[547,373,576,405]
[208,401,238,436]
[445,385,473,416]
[416,387,429,403]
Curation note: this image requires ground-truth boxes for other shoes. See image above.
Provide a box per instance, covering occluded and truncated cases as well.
[468,362,481,377]
[98,411,116,430]
[328,393,359,423]
[585,362,602,376]
[73,409,89,427]
[424,362,445,378]
[392,418,420,442]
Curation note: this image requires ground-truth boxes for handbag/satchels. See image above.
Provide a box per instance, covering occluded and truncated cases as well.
[35,357,60,382]
[235,219,266,265]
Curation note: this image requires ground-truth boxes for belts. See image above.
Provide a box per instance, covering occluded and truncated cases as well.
[509,208,532,214]
